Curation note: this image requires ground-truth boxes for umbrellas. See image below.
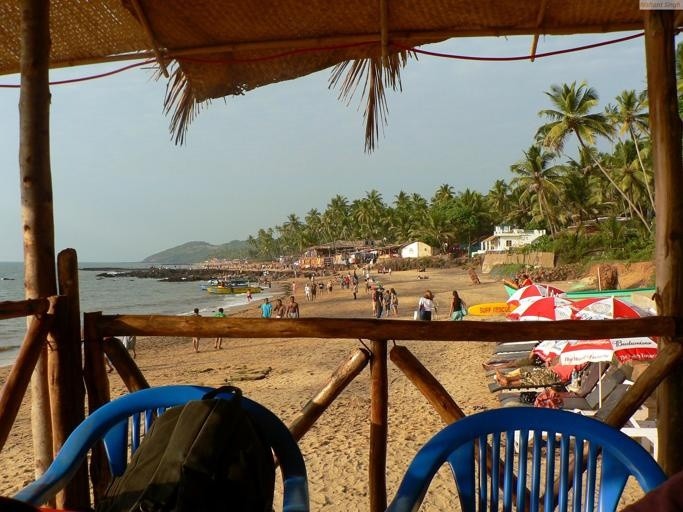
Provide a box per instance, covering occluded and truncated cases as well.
[505,281,655,323]
[532,337,659,410]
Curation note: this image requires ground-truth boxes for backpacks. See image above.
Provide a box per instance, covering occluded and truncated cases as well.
[97,385,276,512]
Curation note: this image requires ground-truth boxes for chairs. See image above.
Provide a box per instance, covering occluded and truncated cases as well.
[11,384,309,512]
[384,407,667,512]
[485,341,633,422]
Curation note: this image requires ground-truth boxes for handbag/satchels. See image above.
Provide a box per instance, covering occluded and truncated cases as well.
[461,304,468,316]
[420,304,425,319]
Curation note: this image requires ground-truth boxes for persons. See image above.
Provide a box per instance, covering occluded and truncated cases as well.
[284,295,299,319]
[102,354,115,374]
[418,289,435,321]
[424,287,439,321]
[448,290,468,322]
[416,276,432,281]
[211,307,226,349]
[190,308,202,353]
[174,258,360,300]
[259,296,273,320]
[274,298,286,318]
[122,336,136,360]
[361,262,400,319]
[513,267,568,287]
[480,343,561,370]
[493,359,593,385]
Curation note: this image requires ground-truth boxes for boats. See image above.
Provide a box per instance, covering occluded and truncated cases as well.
[207,278,263,295]
[199,276,248,290]
